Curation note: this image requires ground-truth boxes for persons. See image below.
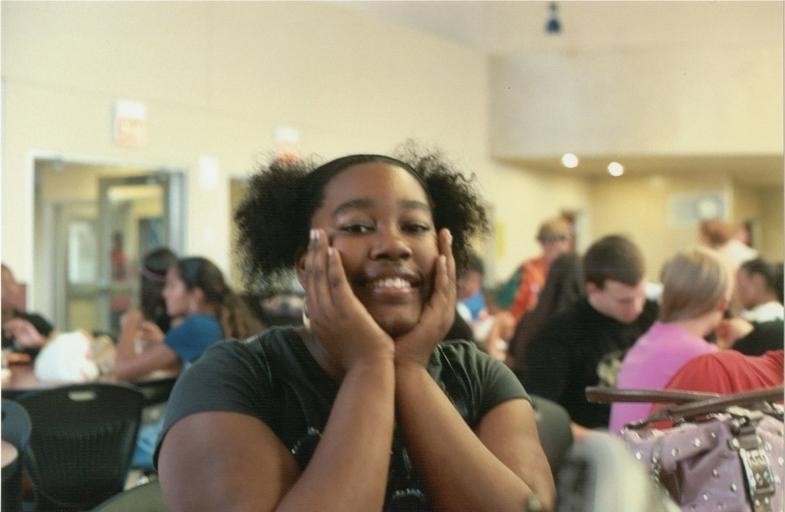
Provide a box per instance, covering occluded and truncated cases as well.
[445,214,785,449]
[156,146,561,511]
[112,255,228,494]
[116,244,177,362]
[1,262,50,365]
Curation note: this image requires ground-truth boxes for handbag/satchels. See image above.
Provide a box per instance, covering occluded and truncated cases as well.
[33,331,99,385]
[619,406,785,512]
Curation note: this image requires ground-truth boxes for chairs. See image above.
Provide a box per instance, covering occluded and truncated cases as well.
[0,381,151,512]
